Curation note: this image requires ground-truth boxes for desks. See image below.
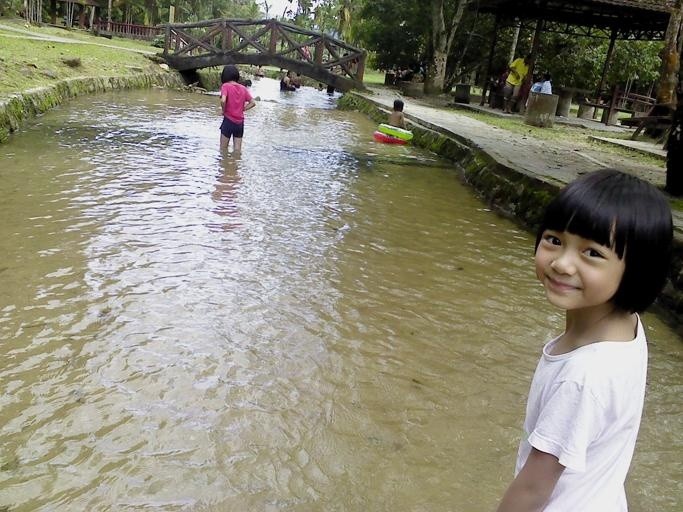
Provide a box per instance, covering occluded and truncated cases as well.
[583,103,634,124]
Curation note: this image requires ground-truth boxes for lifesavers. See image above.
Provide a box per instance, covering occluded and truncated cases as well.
[374,123,414,144]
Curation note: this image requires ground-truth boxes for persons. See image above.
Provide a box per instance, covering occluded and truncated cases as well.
[256,64,264,78]
[524,72,553,107]
[279,69,296,92]
[340,51,350,78]
[385,99,407,129]
[494,165,674,512]
[501,51,536,114]
[313,83,323,91]
[515,70,535,109]
[212,162,245,240]
[293,70,302,88]
[217,64,255,155]
[300,43,312,62]
